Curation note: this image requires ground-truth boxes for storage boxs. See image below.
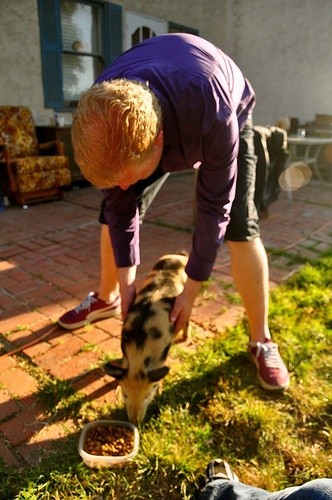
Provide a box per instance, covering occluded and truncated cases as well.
[79,420,140,470]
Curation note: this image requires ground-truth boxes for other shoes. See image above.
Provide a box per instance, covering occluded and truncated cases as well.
[193,458,239,499]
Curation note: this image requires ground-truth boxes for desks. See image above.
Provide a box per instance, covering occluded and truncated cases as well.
[288,137,331,192]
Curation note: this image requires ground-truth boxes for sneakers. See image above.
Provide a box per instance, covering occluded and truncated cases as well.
[58,292,121,330]
[246,337,290,390]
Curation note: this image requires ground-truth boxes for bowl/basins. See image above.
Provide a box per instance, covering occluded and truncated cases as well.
[79,420,138,468]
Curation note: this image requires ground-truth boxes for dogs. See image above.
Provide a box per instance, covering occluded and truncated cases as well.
[99,249,198,421]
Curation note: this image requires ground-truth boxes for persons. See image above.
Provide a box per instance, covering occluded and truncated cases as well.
[57,34,290,391]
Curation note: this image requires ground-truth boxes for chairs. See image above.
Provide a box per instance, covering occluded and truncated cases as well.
[0,104,74,207]
